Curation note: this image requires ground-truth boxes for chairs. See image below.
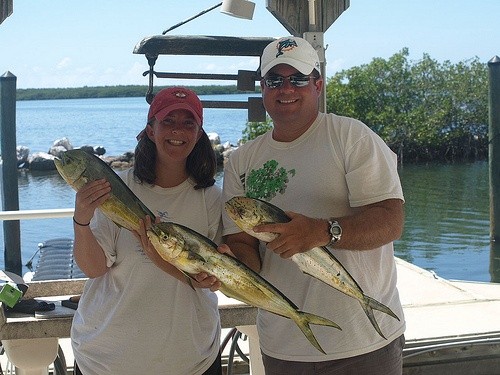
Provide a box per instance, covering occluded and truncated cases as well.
[26,238,74,282]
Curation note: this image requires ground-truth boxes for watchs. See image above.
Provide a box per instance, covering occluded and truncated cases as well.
[323,216,342,248]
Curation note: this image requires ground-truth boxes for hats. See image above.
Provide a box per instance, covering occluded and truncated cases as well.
[147,87,204,126]
[261,35,322,78]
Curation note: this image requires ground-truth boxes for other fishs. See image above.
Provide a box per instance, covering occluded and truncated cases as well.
[147,222,342,356]
[54,150,157,236]
[224,197,401,340]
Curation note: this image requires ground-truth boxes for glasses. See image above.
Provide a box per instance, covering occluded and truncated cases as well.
[264,73,316,87]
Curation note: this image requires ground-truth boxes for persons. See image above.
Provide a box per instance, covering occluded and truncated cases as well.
[72,88,222,375]
[193,37,406,375]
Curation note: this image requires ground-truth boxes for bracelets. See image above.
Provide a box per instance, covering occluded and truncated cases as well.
[73,215,90,226]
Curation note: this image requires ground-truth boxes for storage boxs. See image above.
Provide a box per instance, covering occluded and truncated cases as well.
[0,284,21,308]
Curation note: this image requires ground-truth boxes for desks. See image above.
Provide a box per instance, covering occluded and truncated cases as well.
[0,289,266,375]
